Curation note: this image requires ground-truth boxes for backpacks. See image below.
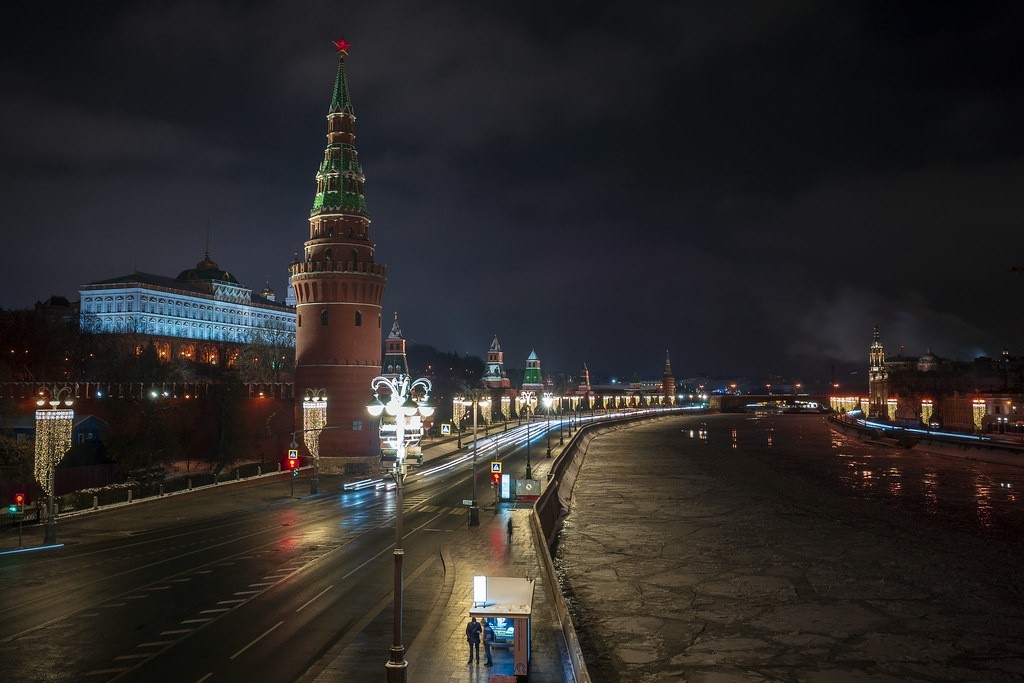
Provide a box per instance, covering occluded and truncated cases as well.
[485,627,496,642]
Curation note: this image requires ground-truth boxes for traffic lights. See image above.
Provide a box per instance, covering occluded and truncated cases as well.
[9,504,15,513]
[15,494,24,514]
[290,459,295,468]
[493,474,500,482]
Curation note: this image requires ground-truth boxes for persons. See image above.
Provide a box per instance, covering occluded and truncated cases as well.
[466,617,482,665]
[480,619,494,667]
[508,518,513,542]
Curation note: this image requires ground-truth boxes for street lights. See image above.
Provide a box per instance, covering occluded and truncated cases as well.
[515,390,537,480]
[35,387,74,544]
[563,394,578,437]
[579,394,707,426]
[304,388,328,494]
[365,373,438,683]
[541,392,560,458]
[452,385,492,527]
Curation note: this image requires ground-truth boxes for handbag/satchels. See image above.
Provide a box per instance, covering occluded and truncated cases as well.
[467,635,470,642]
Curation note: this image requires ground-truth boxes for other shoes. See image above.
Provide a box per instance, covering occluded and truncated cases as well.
[484,662,493,667]
[468,659,473,663]
[476,660,479,665]
